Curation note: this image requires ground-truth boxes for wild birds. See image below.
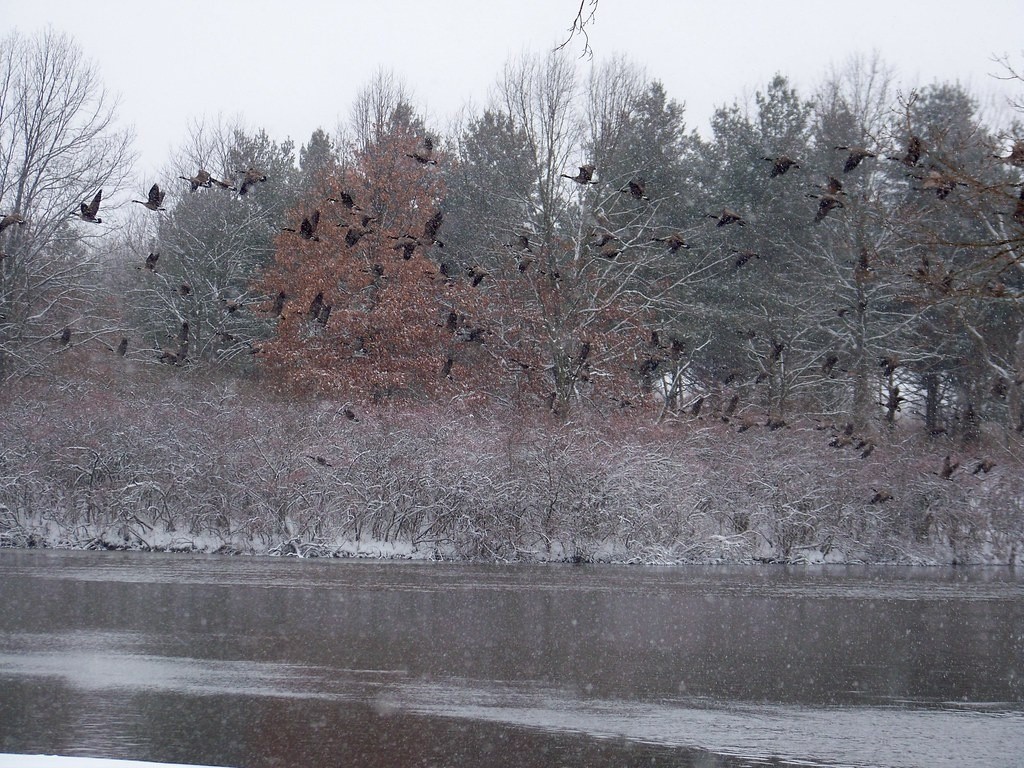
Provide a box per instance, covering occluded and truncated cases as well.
[559,137,1023,505]
[0,138,556,467]
